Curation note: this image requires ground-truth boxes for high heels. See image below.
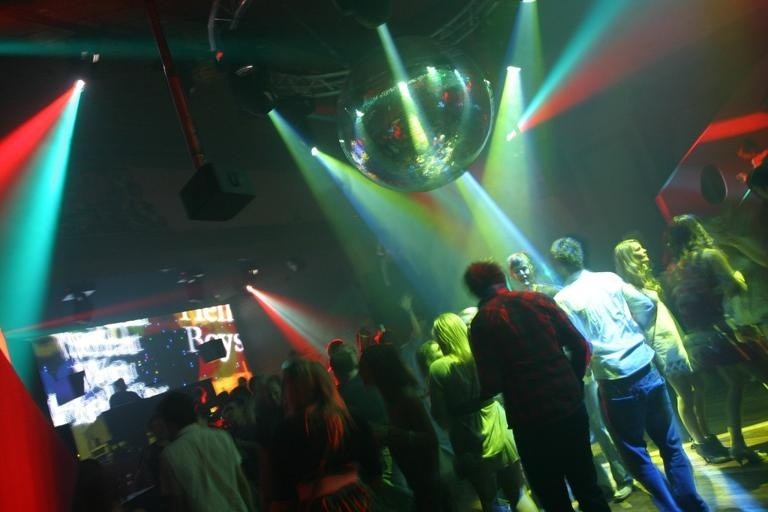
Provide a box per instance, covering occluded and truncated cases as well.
[695,436,728,463]
[731,447,764,466]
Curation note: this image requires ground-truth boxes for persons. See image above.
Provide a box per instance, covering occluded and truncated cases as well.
[110,214,767,512]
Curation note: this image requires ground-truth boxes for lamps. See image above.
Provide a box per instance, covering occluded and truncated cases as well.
[230,58,281,117]
[64,34,106,87]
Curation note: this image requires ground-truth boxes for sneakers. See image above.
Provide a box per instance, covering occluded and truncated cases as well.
[614,485,633,500]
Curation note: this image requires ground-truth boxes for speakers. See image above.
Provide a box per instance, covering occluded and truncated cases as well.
[179,159,259,222]
[236,259,271,283]
